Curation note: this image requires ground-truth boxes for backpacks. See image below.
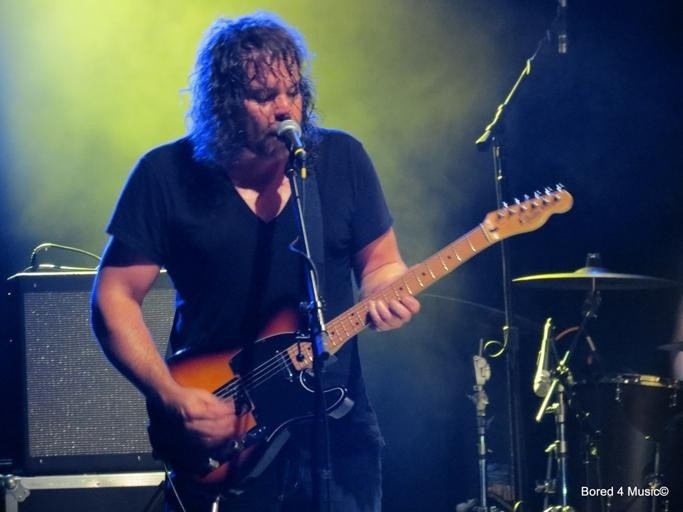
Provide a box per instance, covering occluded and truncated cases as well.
[564,369,682,510]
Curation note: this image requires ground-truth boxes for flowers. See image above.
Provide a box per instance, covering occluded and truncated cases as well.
[274,119,307,161]
[556,1,569,55]
[523,331,553,398]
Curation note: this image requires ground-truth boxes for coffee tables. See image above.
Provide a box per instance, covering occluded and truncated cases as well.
[512,266,682,292]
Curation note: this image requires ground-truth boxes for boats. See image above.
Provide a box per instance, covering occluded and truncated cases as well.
[0,264,177,478]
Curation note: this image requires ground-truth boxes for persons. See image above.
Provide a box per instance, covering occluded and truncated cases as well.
[90,10,421,512]
